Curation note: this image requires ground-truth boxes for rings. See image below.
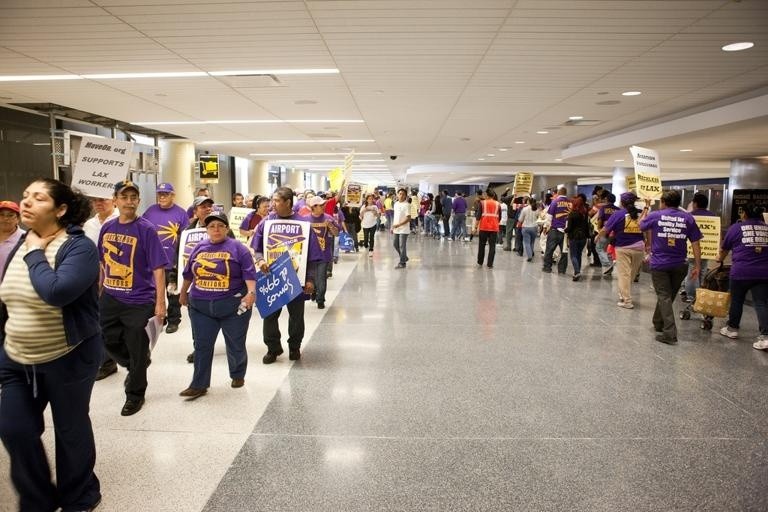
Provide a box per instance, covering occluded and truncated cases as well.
[159,316,165,321]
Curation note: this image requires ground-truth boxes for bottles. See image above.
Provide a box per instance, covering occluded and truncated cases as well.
[237,301,248,316]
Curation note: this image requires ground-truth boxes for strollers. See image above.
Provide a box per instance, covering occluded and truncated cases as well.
[678,260,731,330]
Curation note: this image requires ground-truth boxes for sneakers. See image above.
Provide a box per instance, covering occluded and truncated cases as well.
[617,301,634,309]
[572,262,613,281]
[95,360,117,380]
[369,251,373,256]
[720,326,739,338]
[753,339,768,349]
[317,256,338,309]
[682,297,694,302]
[678,287,688,295]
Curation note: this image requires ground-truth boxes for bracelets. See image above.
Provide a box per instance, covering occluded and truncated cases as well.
[644,206,650,212]
[248,291,256,295]
[254,258,264,265]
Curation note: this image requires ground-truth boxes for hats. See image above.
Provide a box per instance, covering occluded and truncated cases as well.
[203,210,228,224]
[115,179,139,194]
[309,196,328,207]
[0,200,20,212]
[620,192,637,204]
[192,196,214,209]
[155,183,173,193]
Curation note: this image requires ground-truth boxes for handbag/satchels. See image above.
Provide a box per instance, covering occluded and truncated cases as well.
[693,279,731,318]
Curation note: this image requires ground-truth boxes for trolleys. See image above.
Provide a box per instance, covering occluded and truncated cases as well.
[200,160,219,176]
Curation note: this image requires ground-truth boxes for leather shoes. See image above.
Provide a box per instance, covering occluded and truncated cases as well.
[656,335,677,342]
[187,351,194,362]
[263,348,283,364]
[124,359,151,385]
[231,379,244,388]
[289,349,300,360]
[121,395,144,416]
[180,386,207,396]
[166,322,178,333]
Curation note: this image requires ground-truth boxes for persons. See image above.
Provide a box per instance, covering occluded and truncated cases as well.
[374,187,469,241]
[713,202,768,351]
[391,188,412,268]
[340,195,379,258]
[0,176,104,512]
[684,194,717,303]
[293,178,347,310]
[639,189,705,345]
[250,187,322,365]
[184,187,236,364]
[97,180,171,417]
[472,187,650,311]
[179,213,256,398]
[79,192,135,383]
[0,196,28,282]
[141,182,191,335]
[234,193,270,239]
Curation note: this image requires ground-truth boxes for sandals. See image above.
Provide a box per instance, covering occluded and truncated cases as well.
[395,262,406,268]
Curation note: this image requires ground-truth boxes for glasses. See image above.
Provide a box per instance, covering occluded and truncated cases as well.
[89,197,109,202]
